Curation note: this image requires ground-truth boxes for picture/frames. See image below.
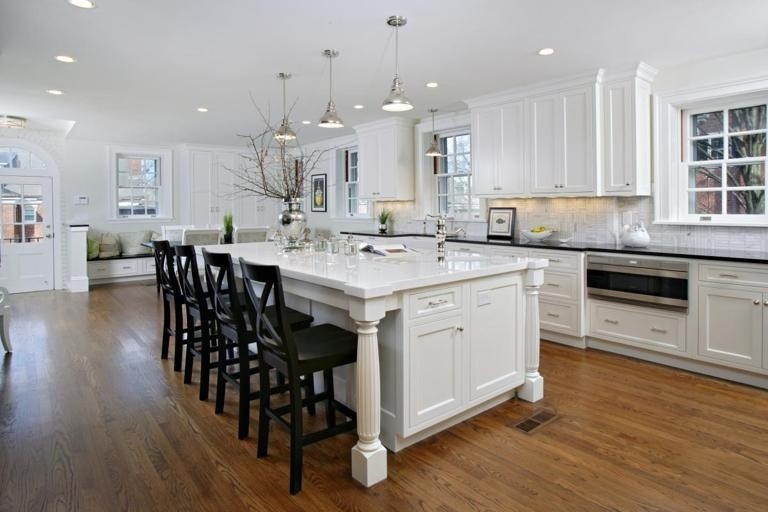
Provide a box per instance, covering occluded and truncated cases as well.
[486,206,517,241]
[309,173,328,213]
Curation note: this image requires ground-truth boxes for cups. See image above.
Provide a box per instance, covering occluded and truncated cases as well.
[559,223,569,243]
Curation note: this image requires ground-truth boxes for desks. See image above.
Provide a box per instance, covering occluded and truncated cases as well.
[166,237,550,488]
[140,241,183,295]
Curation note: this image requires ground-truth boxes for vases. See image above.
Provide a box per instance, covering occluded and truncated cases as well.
[374,208,393,233]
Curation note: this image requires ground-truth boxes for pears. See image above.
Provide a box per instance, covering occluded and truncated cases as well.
[530,223,545,232]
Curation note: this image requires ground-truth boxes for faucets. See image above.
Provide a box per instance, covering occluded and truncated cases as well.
[424,213,442,228]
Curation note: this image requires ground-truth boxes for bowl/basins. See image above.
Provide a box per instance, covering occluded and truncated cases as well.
[522,230,552,242]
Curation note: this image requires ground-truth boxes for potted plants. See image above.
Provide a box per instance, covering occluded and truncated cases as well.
[222,211,233,244]
[213,87,347,244]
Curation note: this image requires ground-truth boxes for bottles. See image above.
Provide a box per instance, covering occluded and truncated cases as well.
[274,228,358,256]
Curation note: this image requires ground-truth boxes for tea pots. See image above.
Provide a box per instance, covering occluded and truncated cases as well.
[623,222,650,247]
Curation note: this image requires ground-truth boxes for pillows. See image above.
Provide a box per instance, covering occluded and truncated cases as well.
[86,232,100,260]
[116,230,154,258]
[99,232,120,260]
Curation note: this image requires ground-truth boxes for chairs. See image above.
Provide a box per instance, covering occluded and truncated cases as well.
[240,256,360,495]
[199,247,316,418]
[174,243,262,384]
[153,240,229,362]
[162,221,271,247]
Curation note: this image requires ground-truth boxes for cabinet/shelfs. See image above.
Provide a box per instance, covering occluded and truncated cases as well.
[588,300,688,380]
[530,86,595,196]
[472,101,526,196]
[605,79,652,199]
[239,153,280,232]
[179,150,239,229]
[443,241,585,350]
[88,253,175,286]
[689,262,768,392]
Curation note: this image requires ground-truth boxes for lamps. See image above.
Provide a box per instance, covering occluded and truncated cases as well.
[379,14,416,114]
[1,117,29,130]
[271,72,297,142]
[317,48,345,131]
[424,107,443,157]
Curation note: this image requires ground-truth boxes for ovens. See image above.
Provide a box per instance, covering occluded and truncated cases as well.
[582,256,690,314]
